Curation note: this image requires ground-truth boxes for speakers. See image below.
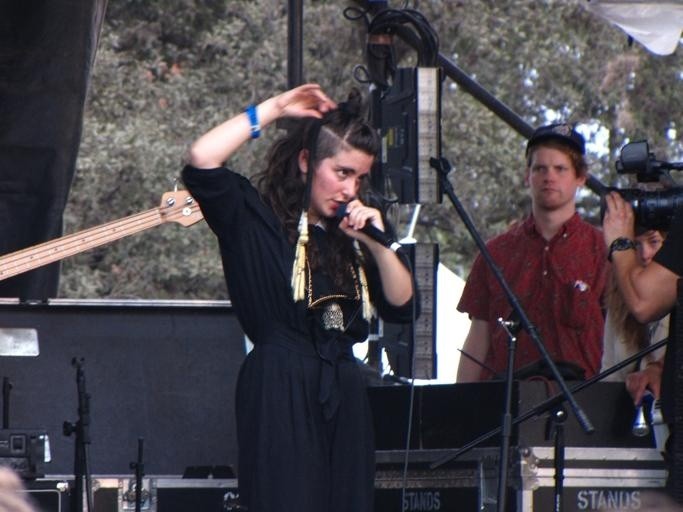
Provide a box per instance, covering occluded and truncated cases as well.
[375,65,442,205]
[367,378,655,447]
[381,238,438,380]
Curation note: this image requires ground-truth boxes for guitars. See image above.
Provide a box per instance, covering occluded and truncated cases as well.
[0,185,205,281]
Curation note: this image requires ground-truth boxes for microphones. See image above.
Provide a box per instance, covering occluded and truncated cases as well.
[335,202,402,252]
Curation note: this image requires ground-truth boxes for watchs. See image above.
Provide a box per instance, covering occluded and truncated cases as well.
[606,236,638,264]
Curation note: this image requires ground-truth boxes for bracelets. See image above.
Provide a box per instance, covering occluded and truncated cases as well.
[243,103,260,139]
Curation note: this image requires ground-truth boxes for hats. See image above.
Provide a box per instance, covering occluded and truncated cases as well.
[524,123,586,158]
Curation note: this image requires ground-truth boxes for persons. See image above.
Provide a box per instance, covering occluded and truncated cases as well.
[180,76,424,510]
[452,117,615,383]
[597,189,681,507]
[587,217,680,376]
